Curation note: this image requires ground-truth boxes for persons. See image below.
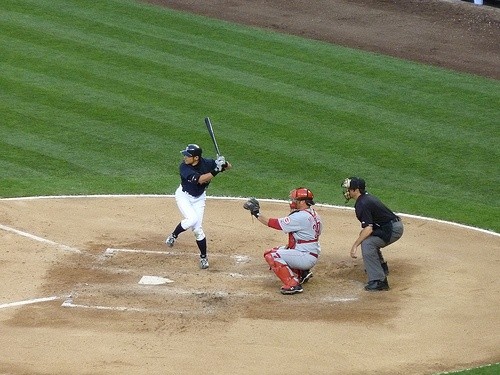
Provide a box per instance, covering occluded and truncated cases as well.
[243,188,322,295]
[166,143,232,269]
[341,176,403,291]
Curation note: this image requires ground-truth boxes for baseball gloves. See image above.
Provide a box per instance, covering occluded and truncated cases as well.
[243,197,260,214]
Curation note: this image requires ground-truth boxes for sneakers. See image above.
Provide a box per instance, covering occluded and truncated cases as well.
[297,269,313,284]
[281,284,303,294]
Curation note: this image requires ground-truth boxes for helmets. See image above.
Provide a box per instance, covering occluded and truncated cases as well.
[342,177,367,200]
[180,144,202,157]
[288,187,315,209]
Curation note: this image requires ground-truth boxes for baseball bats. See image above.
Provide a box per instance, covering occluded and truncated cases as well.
[204,117,221,157]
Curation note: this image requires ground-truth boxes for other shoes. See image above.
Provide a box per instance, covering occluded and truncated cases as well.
[365,276,389,290]
[166,233,178,248]
[381,262,389,274]
[200,255,211,269]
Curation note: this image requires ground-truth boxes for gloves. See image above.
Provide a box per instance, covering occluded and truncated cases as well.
[217,154,228,168]
[211,159,225,177]
